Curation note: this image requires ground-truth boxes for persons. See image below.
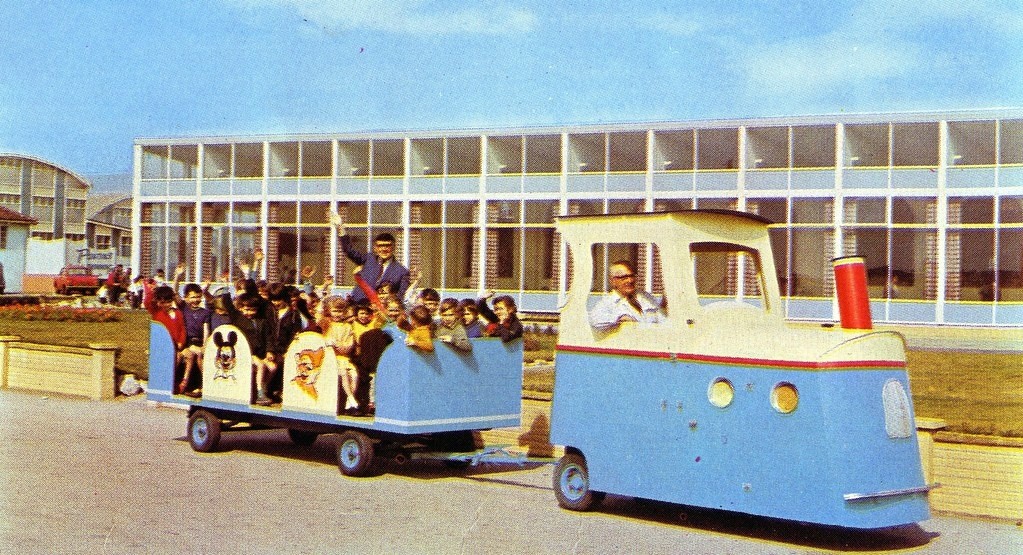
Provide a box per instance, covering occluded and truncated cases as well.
[883,272,900,299]
[588,262,669,333]
[140,212,524,418]
[98,264,166,311]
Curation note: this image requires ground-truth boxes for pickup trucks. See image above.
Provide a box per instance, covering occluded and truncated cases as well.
[52,266,99,296]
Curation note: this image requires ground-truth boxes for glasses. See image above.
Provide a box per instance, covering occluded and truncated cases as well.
[613,272,636,280]
[375,243,392,248]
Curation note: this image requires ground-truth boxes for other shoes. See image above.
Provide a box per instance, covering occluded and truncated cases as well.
[365,402,374,415]
[175,381,183,394]
[341,402,351,412]
[352,402,360,413]
[254,396,271,403]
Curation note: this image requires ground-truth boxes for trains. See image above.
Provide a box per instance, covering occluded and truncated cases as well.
[143,206,940,535]
